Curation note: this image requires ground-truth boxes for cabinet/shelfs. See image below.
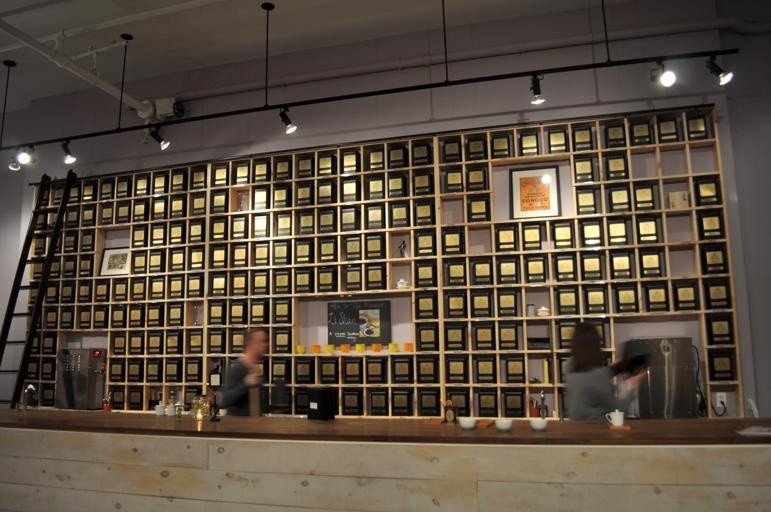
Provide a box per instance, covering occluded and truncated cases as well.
[21,104,745,418]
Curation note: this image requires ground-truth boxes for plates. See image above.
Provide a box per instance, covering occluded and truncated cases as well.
[396,287,410,289]
[536,313,550,316]
[736,430,770,439]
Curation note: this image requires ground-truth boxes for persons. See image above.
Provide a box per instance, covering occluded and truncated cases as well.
[222,327,269,416]
[562,322,653,422]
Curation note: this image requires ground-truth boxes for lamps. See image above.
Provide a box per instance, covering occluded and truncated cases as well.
[7,1,735,171]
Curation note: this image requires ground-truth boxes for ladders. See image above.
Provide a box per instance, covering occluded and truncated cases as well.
[0,169,77,408]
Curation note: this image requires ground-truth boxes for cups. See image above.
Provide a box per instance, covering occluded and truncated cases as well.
[536,306,548,313]
[154,400,184,421]
[296,342,414,355]
[395,281,408,286]
[605,408,625,427]
[218,409,228,416]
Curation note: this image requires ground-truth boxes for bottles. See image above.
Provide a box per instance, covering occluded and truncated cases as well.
[526,303,535,317]
[102,398,111,416]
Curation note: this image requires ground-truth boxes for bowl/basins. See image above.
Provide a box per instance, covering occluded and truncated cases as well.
[459,416,549,432]
